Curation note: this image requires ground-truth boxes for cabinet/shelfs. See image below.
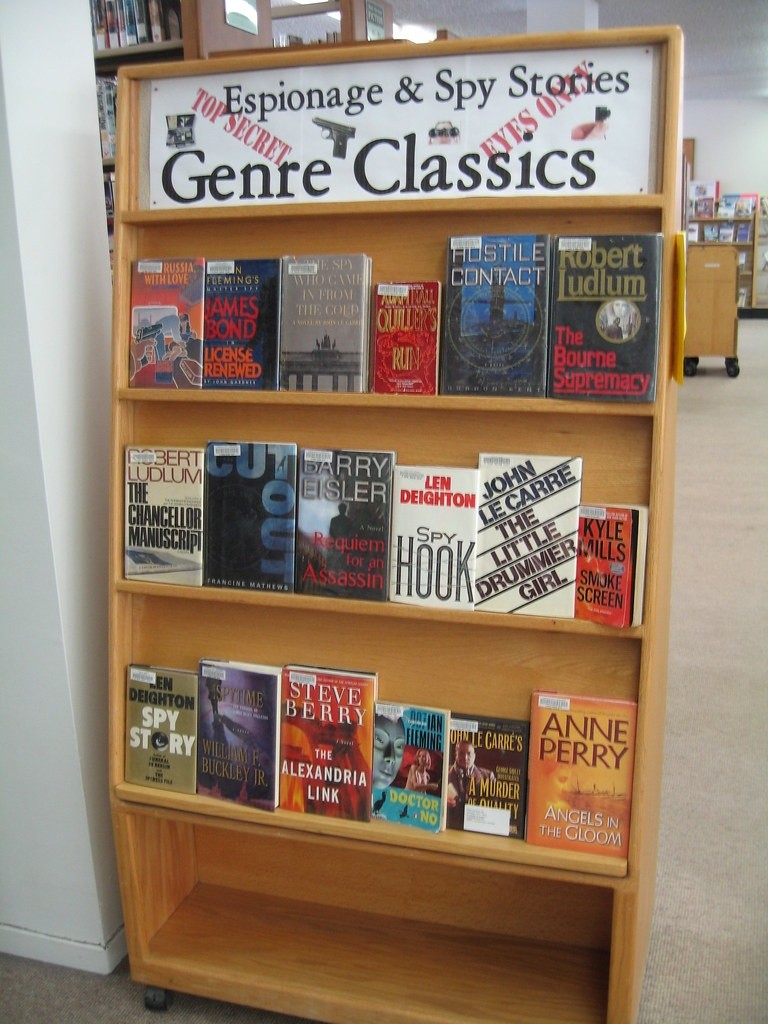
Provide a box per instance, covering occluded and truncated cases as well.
[89,0,768,1024]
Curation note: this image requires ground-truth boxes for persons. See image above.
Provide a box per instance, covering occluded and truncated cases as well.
[607,299,633,321]
[88,1,182,228]
[603,316,624,340]
[371,709,405,793]
[330,503,353,548]
[205,676,222,714]
[406,747,438,794]
[447,740,498,815]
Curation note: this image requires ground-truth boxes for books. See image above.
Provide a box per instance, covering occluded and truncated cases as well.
[685,174,757,308]
[274,31,343,47]
[128,232,660,407]
[122,440,649,632]
[123,657,638,865]
